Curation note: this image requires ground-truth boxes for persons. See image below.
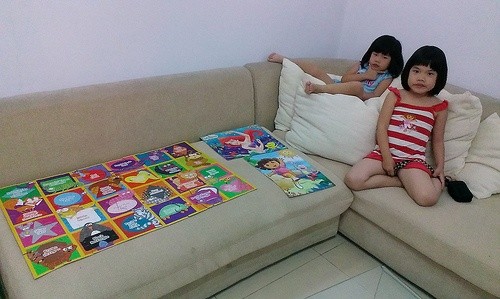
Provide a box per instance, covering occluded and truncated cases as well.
[345,46,459,207]
[268,35,404,101]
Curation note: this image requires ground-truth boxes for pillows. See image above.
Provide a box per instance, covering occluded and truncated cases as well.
[274,57,500,200]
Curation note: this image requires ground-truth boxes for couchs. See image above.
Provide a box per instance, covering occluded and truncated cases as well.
[0,58,500,299]
